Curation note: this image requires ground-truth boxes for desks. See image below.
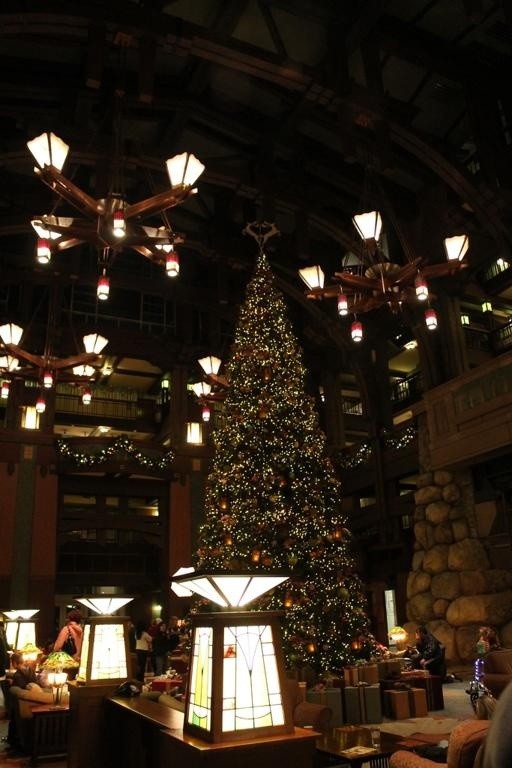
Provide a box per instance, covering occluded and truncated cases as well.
[315,716,465,768]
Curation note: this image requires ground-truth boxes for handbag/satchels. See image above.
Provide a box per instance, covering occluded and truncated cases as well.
[62,637,77,655]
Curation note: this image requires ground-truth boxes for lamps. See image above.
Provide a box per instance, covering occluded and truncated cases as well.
[169,568,295,742]
[298,210,512,351]
[1,607,41,665]
[387,626,409,646]
[65,591,142,699]
[25,131,204,301]
[0,324,222,447]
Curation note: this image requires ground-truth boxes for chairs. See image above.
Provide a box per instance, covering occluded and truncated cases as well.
[389,720,492,768]
[484,650,512,688]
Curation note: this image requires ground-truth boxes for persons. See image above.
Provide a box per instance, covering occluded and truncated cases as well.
[391,628,446,676]
[129,616,188,682]
[476,626,502,655]
[0,609,83,689]
